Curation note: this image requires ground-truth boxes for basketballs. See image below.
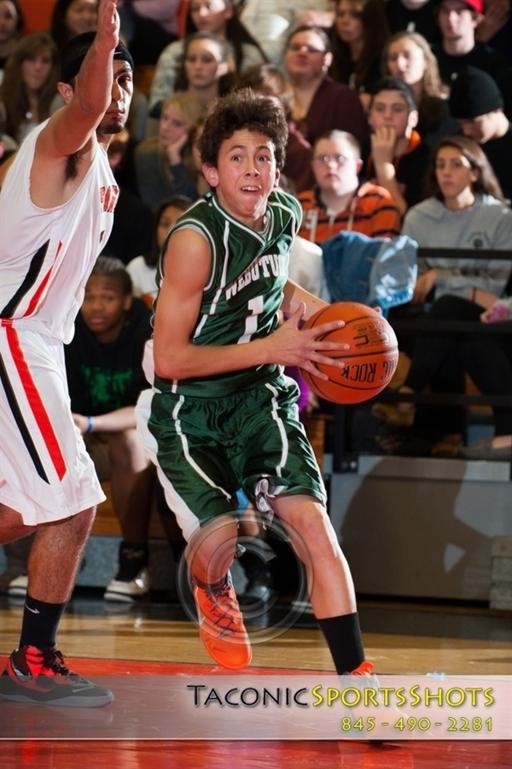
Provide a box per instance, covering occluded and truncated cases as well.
[299,303,398,405]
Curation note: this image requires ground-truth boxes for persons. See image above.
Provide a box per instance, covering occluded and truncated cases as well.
[133,86,414,743]
[0,1,511,629]
[0,0,136,708]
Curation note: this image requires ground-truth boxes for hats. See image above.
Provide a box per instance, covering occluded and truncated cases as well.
[450,65,503,118]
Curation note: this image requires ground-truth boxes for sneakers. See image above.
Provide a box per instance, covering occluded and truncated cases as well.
[190,571,251,671]
[104,566,176,605]
[1,647,113,707]
[7,573,30,597]
